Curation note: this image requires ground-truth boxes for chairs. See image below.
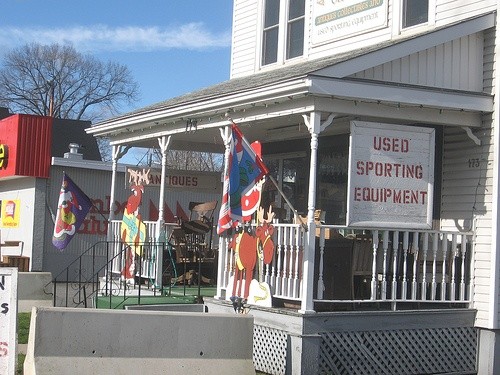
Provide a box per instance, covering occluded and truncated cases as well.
[330,234,381,311]
[167,200,219,288]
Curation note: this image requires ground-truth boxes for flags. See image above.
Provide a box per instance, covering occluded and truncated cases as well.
[52,173,92,251]
[217,125,269,234]
